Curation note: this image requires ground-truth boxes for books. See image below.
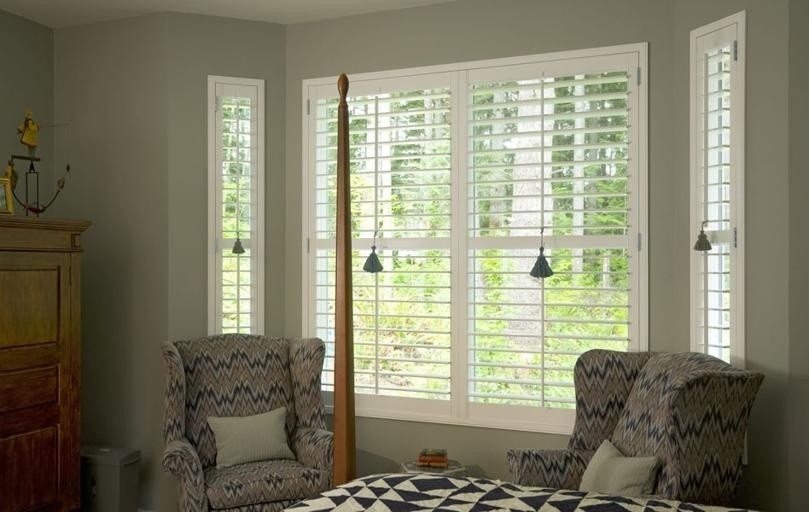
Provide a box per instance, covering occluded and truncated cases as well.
[417,448,448,467]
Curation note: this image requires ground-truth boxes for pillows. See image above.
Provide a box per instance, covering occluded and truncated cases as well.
[205,405,298,471]
[576,438,660,497]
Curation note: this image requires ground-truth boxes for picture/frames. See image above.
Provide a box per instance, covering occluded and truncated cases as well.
[0,177,14,214]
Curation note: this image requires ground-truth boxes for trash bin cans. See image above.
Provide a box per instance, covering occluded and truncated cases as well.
[80,444,140,512]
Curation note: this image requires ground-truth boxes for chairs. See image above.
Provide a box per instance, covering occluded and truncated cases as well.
[159,333,335,512]
[505,349,766,505]
